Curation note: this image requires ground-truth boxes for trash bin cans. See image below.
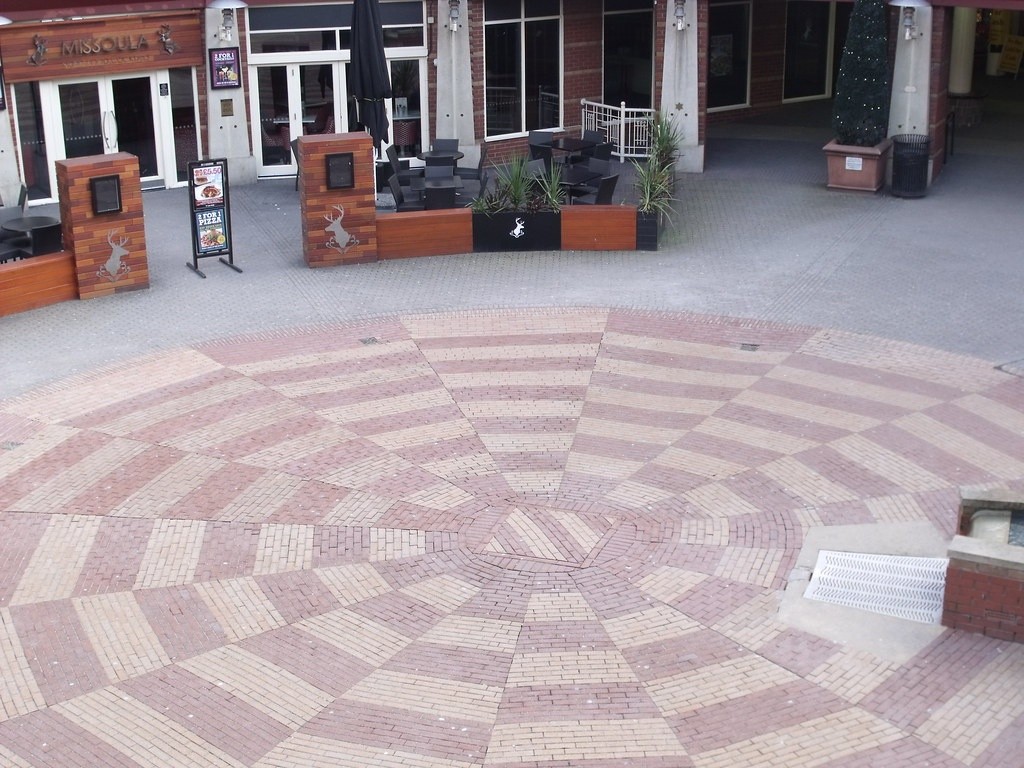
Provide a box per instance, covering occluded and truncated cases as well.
[890,134,933,198]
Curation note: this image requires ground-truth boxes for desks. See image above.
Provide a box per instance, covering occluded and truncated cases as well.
[272,111,317,124]
[540,137,597,152]
[394,109,421,120]
[409,175,465,202]
[417,151,464,161]
[542,166,602,204]
[1,216,59,232]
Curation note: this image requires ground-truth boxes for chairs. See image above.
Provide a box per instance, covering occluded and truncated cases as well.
[394,121,419,157]
[452,142,487,184]
[388,173,427,213]
[261,124,283,165]
[455,171,490,207]
[432,138,460,153]
[289,139,301,192]
[280,126,308,164]
[309,115,336,134]
[424,165,453,176]
[386,144,424,201]
[520,130,619,205]
[0,185,62,264]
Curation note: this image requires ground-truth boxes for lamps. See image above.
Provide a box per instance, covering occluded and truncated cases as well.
[448,0,461,32]
[672,0,687,32]
[889,1,932,41]
[207,0,248,41]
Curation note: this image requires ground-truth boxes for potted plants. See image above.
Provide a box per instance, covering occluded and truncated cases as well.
[822,0,894,195]
[464,147,568,252]
[625,106,687,251]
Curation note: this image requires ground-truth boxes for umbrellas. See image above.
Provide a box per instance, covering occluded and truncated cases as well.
[350,0,394,158]
